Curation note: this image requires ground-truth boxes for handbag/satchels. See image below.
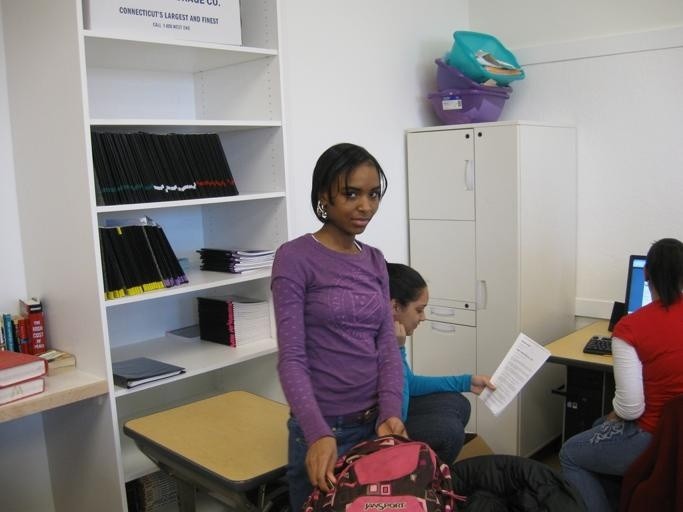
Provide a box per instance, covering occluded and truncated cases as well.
[304,435,455,512]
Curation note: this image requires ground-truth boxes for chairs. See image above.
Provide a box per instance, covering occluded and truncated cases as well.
[582,388,681,512]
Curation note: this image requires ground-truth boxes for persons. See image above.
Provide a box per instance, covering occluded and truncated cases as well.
[558,238,682,511]
[387,262,496,468]
[271,142,409,510]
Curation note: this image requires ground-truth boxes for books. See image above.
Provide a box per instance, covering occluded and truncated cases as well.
[0,297,76,406]
[198,295,275,347]
[196,247,275,274]
[92,130,239,206]
[98,218,190,300]
[168,325,199,340]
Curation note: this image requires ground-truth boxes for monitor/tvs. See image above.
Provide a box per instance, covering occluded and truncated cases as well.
[623,255,653,315]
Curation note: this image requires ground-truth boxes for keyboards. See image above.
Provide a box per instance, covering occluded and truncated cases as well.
[583,335,612,355]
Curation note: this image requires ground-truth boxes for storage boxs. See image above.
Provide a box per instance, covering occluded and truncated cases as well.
[426,30,526,125]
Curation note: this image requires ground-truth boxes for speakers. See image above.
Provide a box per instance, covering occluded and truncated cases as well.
[608,301,625,332]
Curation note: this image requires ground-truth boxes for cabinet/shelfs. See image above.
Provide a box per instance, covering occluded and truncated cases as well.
[407,122,579,459]
[1,0,289,512]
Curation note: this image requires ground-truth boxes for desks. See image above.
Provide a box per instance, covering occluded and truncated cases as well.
[122,389,292,511]
[0,366,108,428]
[539,318,606,449]
